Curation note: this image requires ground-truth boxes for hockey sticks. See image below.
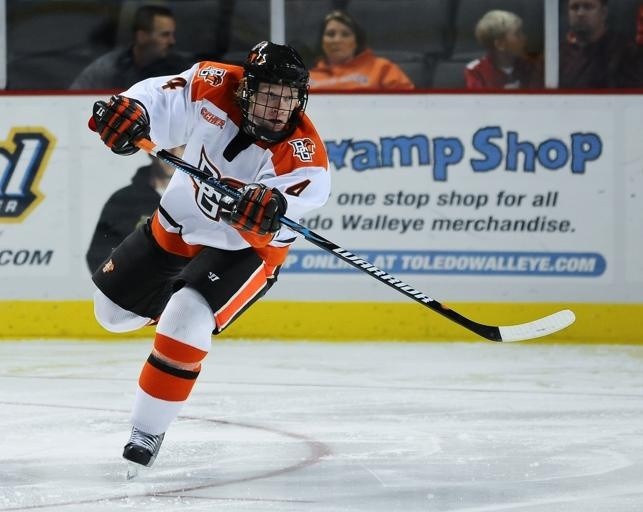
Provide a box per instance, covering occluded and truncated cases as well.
[93,102,576,343]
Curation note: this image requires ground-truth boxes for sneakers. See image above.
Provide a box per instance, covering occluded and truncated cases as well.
[122,425,165,467]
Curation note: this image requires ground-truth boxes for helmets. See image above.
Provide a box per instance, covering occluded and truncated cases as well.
[235,41,311,145]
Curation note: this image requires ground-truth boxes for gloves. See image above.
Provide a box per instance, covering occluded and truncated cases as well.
[86,95,151,159]
[217,182,288,236]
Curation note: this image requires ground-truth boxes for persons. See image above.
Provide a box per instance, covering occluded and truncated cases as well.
[304,12,416,91]
[86,145,184,276]
[88,40,329,469]
[559,0,643,89]
[68,6,190,90]
[464,9,543,89]
